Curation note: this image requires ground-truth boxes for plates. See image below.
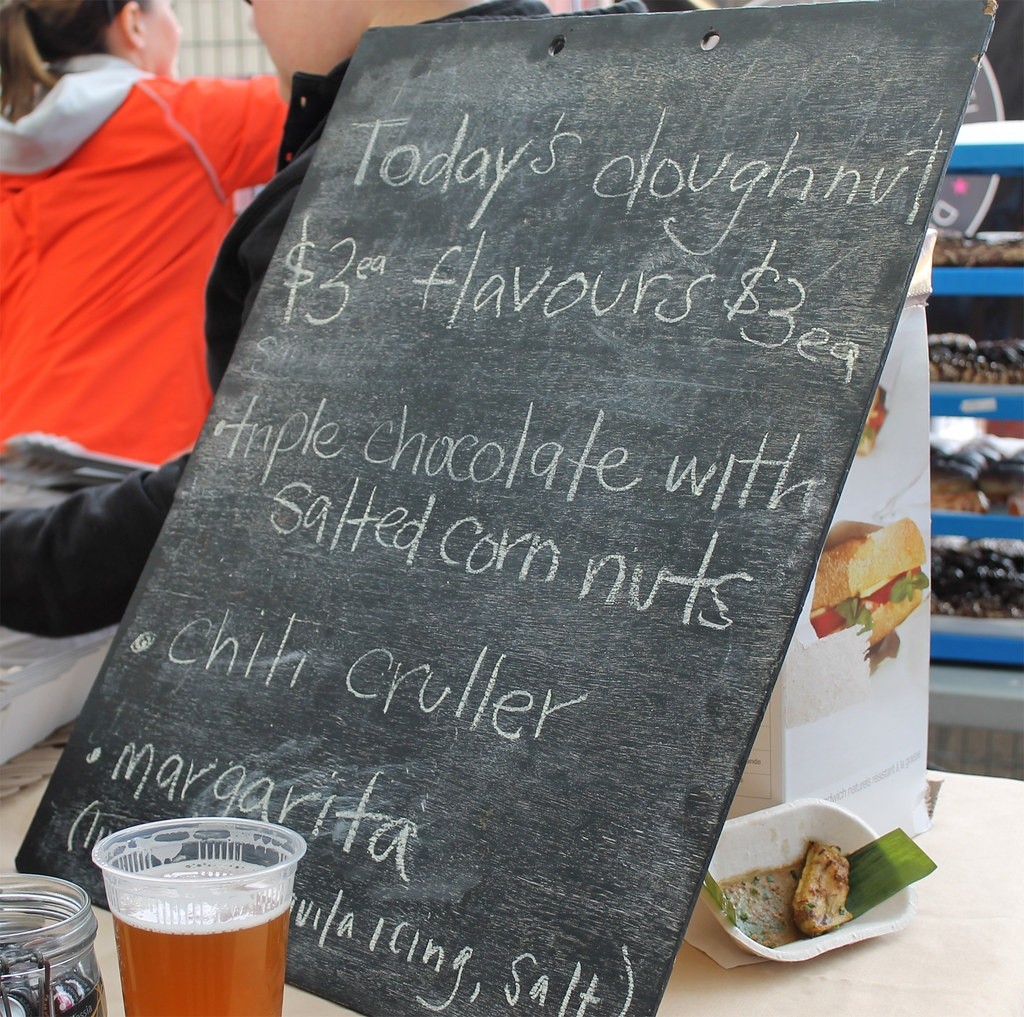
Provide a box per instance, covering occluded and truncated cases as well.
[697,798,916,963]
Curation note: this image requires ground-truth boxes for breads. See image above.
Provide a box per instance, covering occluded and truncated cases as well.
[925,234,1024,623]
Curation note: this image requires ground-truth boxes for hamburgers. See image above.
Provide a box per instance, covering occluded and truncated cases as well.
[811,518,930,650]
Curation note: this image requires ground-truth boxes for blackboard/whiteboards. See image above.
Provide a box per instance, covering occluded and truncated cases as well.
[13,0,1007,1017]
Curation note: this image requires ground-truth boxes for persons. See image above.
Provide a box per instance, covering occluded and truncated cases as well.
[0,0,650,639]
[0,0,289,466]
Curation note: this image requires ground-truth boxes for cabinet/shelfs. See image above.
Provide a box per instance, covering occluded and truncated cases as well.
[929,141,1024,731]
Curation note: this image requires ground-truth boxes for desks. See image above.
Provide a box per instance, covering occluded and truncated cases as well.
[0,767,1024,1017]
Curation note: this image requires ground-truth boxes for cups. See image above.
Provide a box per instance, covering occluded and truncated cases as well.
[90,816,307,1017]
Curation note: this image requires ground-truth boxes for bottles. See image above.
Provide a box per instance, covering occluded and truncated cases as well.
[0,873,110,1017]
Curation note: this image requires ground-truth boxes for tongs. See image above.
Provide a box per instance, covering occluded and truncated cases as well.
[1,434,159,488]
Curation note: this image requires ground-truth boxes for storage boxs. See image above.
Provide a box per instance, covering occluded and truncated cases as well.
[726,227,939,837]
